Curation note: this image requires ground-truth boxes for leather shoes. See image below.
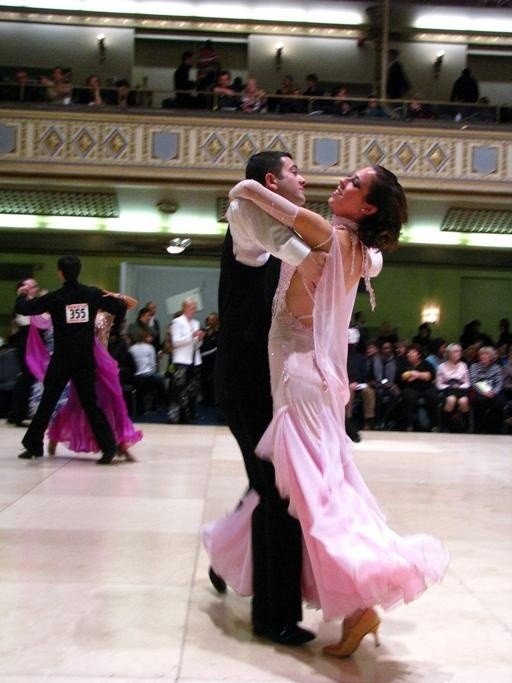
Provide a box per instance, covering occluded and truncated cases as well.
[254,624,316,646]
[18,449,43,458]
[97,448,116,464]
[210,566,226,593]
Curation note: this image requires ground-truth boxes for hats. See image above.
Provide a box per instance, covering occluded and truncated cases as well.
[348,328,360,344]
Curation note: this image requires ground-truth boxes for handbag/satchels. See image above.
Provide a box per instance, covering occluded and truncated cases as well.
[473,379,493,393]
[443,379,468,399]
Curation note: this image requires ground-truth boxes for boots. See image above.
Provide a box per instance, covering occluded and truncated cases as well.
[432,409,451,432]
[458,410,470,433]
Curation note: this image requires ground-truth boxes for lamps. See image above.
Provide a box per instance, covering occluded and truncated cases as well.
[164,238,192,254]
[274,42,283,69]
[96,32,106,58]
[436,50,444,72]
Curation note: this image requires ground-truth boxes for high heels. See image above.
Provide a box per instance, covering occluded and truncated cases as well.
[49,440,57,455]
[322,609,381,657]
[117,446,134,461]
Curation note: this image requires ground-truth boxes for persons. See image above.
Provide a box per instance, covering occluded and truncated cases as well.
[202,164,451,662]
[1,254,220,466]
[0,39,491,121]
[346,317,512,443]
[208,149,337,647]
